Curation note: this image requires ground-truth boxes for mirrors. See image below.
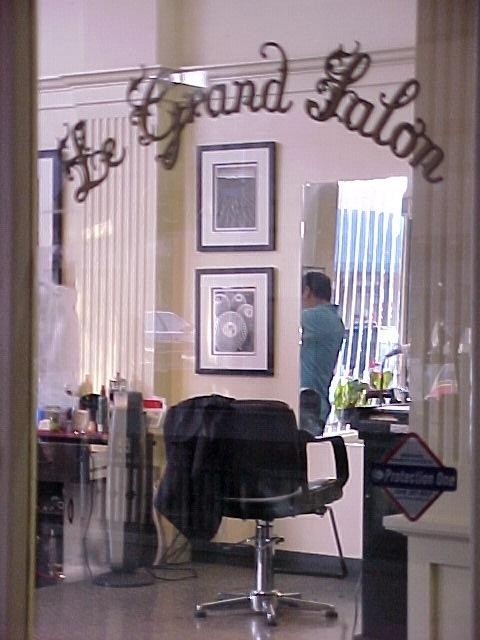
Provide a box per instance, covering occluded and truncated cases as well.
[299,174,408,447]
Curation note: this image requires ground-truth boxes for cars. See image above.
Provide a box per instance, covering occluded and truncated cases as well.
[142,309,195,372]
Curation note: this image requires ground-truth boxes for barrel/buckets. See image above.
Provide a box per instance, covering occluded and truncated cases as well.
[39,523,64,574]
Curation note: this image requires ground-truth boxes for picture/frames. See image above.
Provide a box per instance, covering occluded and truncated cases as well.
[196,141,275,251]
[37,150,62,287]
[194,266,275,375]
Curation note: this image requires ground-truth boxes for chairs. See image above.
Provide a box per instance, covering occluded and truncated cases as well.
[175,398,349,640]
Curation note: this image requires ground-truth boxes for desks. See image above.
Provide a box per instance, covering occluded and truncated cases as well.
[36,426,157,582]
[346,414,409,640]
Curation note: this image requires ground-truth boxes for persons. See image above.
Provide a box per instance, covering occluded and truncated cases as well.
[303,271,345,435]
